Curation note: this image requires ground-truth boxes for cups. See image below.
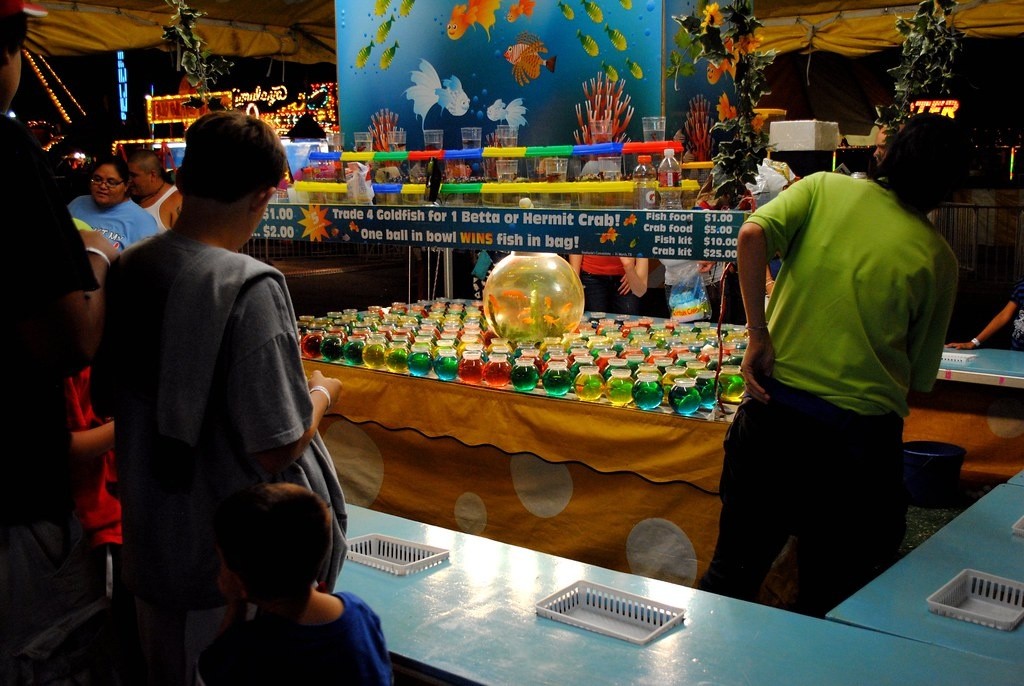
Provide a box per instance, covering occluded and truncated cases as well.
[590,120,612,144]
[423,129,443,150]
[354,132,373,152]
[497,124,518,147]
[460,127,482,149]
[641,117,666,142]
[329,133,344,152]
[387,131,407,151]
[597,156,621,182]
[545,158,568,181]
[496,160,518,183]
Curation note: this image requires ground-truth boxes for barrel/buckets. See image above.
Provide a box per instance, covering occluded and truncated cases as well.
[905,440,968,509]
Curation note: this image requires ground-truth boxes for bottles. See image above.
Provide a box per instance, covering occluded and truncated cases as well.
[632,156,656,210]
[296,252,748,415]
[657,148,683,210]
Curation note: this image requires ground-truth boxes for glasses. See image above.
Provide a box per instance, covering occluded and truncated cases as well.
[90,175,127,188]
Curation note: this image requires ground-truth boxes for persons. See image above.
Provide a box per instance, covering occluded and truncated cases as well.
[64,155,159,253]
[124,150,182,232]
[569,254,782,318]
[0,0,119,685]
[873,124,905,163]
[92,111,342,686]
[193,483,393,685]
[947,278,1024,350]
[700,113,972,613]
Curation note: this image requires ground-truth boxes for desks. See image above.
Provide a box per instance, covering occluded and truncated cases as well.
[301,353,734,590]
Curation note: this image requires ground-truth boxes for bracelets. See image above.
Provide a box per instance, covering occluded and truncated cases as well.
[971,338,980,347]
[766,281,774,286]
[141,181,165,204]
[86,248,110,267]
[310,386,330,408]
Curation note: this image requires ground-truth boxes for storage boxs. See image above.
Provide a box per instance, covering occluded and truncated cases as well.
[770,120,838,150]
